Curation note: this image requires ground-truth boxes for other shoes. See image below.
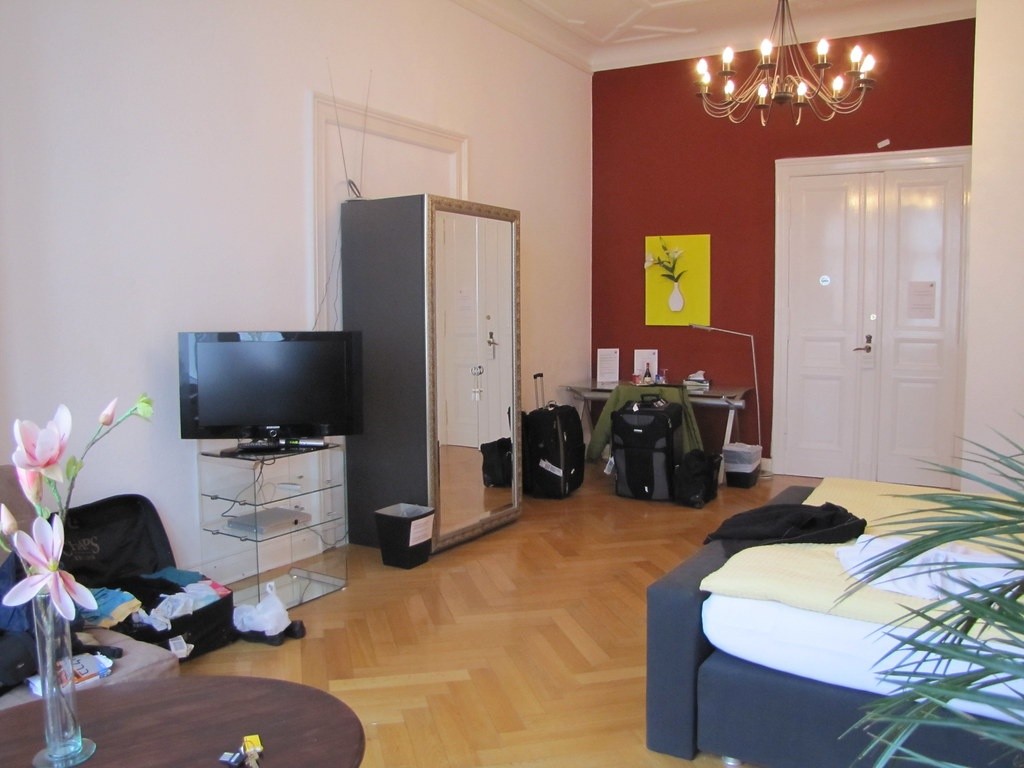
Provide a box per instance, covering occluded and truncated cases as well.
[236,630,285,645]
[284,621,307,639]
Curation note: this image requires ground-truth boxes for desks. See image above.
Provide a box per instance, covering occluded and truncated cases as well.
[0,675,366,768]
[559,378,747,485]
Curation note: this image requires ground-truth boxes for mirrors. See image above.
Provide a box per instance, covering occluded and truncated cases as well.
[433,209,517,533]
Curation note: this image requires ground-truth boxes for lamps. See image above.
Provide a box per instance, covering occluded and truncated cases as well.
[693,0,877,126]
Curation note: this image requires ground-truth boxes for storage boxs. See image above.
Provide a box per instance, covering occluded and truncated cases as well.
[722,443,763,487]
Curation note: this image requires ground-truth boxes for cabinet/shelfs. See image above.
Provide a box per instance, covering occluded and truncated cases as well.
[339,191,522,558]
[199,441,348,612]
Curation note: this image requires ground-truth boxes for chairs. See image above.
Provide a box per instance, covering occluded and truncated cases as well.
[0,462,181,708]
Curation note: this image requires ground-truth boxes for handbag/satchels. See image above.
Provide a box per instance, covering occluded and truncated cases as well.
[479,437,511,488]
[672,449,718,509]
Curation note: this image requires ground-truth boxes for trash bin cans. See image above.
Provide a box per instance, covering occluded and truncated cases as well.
[373,503,437,569]
[723,443,763,487]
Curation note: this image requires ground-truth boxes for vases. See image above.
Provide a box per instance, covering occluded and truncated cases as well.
[31,592,83,760]
[670,282,684,311]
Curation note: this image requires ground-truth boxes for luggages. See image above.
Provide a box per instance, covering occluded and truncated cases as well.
[526,372,586,500]
[610,393,682,502]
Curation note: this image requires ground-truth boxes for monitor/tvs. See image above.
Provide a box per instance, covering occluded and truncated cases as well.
[178,331,365,460]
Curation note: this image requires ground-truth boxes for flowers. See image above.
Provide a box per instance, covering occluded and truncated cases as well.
[643,235,688,283]
[0,392,157,725]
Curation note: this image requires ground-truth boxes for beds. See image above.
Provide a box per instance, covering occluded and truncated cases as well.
[645,477,1024,768]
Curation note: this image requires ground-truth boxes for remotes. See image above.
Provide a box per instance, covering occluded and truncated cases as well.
[239,441,280,450]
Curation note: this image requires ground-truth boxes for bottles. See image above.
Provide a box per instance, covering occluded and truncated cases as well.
[644,363,652,383]
[664,368,671,384]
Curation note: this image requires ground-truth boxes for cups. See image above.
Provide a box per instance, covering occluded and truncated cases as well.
[632,374,640,384]
